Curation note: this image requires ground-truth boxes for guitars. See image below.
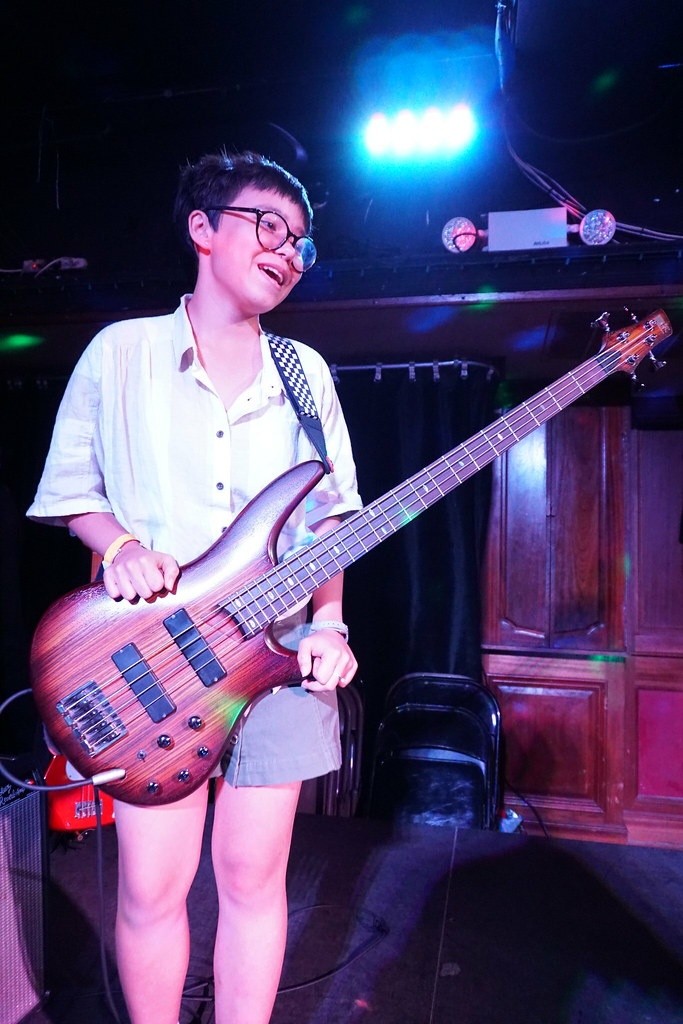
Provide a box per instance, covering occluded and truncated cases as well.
[24,301,677,809]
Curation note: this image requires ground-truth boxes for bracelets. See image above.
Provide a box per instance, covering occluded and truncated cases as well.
[102,534,140,569]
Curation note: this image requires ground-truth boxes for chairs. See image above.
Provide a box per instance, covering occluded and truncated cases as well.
[295,672,502,827]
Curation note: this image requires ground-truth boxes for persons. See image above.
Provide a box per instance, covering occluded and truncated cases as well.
[25,155,358,1024]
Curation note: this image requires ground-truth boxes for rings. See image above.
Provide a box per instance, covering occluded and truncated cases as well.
[333,672,343,683]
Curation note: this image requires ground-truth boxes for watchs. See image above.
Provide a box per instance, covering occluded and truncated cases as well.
[308,621,349,642]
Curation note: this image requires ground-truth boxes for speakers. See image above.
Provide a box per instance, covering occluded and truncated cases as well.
[0,765,46,1024]
